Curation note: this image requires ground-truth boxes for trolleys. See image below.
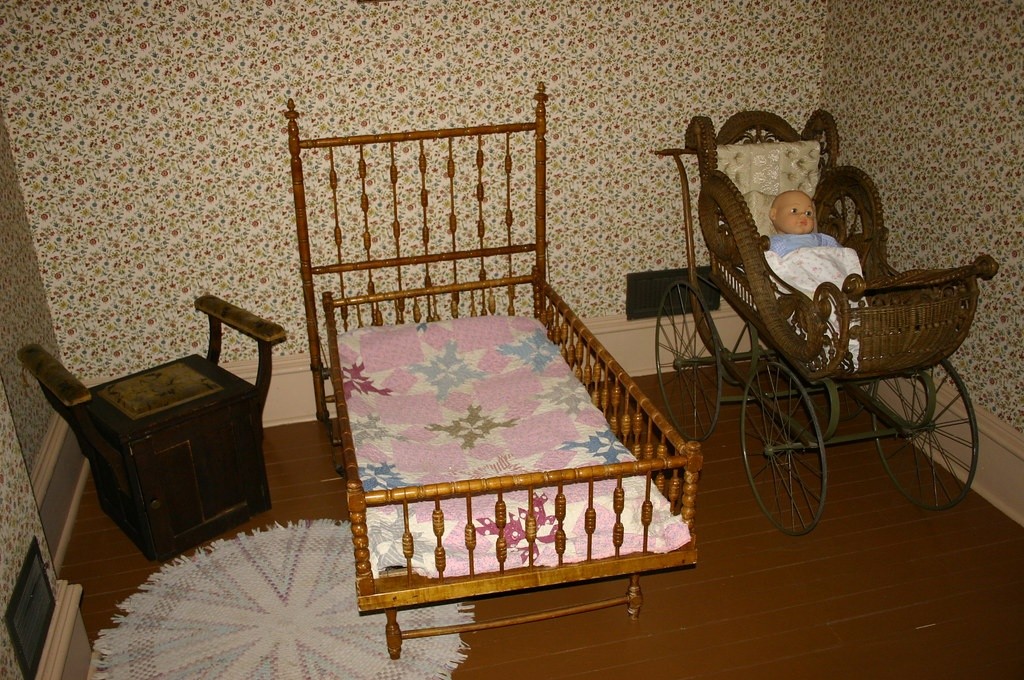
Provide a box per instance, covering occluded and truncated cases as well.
[649,108,1006,535]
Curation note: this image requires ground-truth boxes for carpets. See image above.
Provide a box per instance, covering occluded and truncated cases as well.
[92,518,476,680]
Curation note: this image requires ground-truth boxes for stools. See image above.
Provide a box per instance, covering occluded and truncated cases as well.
[17,295,288,562]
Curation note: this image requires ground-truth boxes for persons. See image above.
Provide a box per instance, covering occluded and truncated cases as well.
[767,190,843,258]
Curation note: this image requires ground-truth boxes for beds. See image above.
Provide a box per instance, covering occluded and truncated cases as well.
[285,81,705,659]
